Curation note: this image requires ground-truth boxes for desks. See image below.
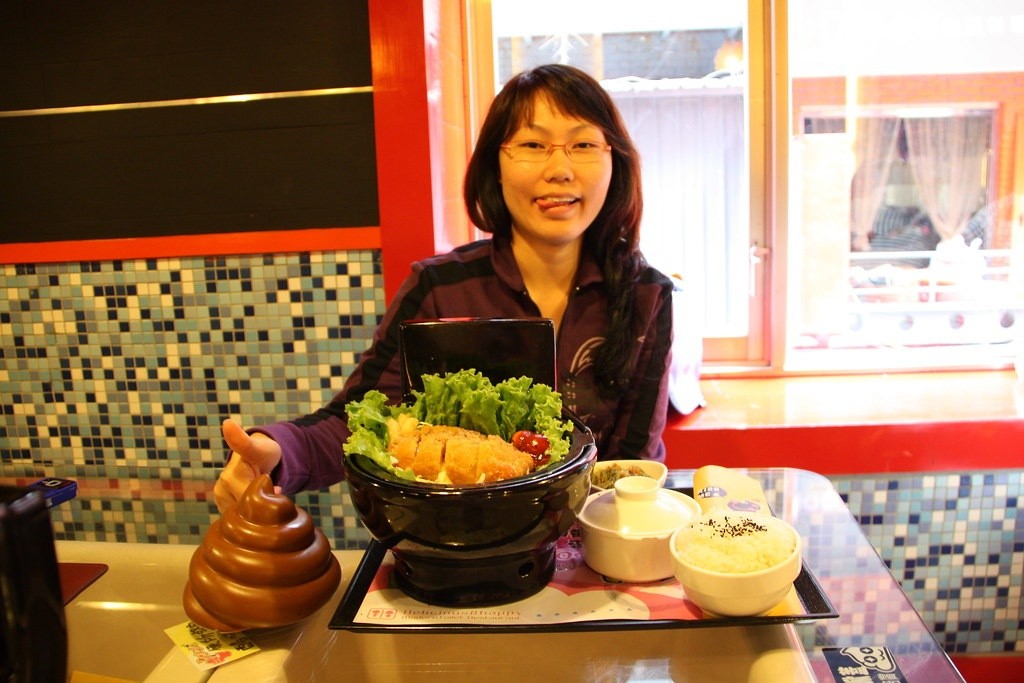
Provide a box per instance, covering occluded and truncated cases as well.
[0,468,970,683]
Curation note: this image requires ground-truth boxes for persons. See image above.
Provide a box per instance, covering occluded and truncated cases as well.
[214,64,676,514]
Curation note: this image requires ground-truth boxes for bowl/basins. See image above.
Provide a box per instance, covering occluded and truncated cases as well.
[591,459,668,492]
[670,512,801,619]
[342,402,597,563]
[575,487,702,583]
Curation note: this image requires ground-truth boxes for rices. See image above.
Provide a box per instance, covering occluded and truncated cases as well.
[674,506,793,574]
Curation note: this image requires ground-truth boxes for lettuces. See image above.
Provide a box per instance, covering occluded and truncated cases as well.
[342,368,574,482]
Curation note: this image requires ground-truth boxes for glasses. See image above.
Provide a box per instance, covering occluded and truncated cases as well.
[500,140,611,162]
